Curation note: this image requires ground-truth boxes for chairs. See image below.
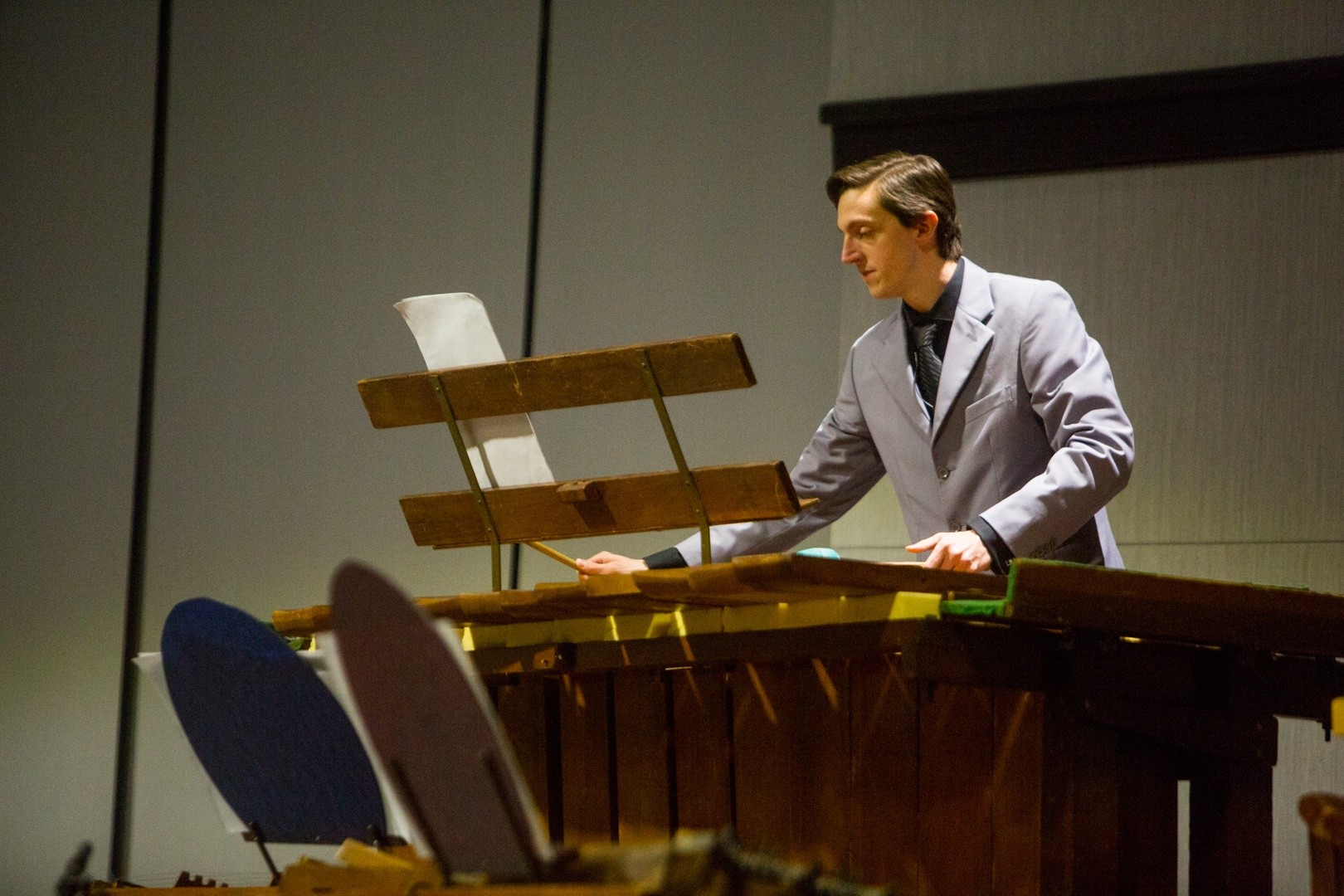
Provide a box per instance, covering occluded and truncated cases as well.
[326,559,604,886]
[161,596,408,888]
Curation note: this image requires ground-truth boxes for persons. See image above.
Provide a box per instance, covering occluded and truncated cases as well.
[576,152,1133,578]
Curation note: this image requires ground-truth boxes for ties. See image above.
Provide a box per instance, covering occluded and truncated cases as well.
[911,323,942,428]
